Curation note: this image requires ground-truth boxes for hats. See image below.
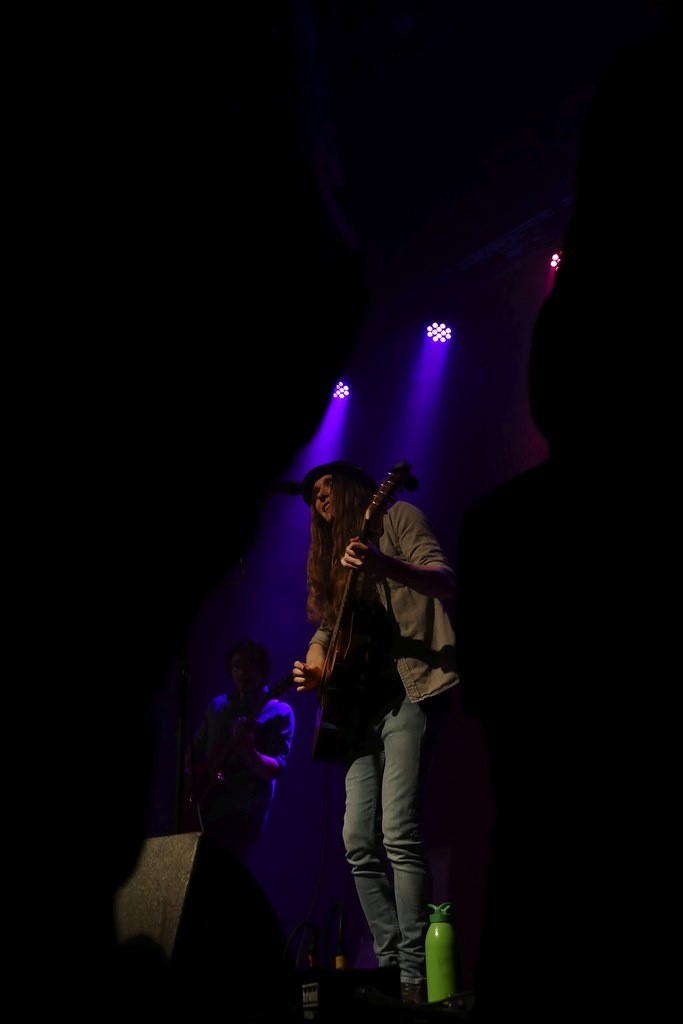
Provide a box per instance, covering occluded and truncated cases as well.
[301,461,385,506]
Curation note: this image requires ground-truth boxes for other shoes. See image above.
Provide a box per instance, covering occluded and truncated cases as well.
[399,980,423,1006]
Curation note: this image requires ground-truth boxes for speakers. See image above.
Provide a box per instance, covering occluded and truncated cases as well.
[110,831,295,991]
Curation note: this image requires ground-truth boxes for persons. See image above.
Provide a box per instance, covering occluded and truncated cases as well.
[182,641,295,977]
[291,459,460,1007]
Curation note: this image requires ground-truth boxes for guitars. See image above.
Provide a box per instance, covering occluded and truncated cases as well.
[308,460,408,769]
[177,671,296,826]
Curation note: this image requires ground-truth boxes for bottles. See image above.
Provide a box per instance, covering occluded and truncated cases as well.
[424,902,457,1003]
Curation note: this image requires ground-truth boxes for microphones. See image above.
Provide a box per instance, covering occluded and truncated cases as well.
[264,480,302,495]
